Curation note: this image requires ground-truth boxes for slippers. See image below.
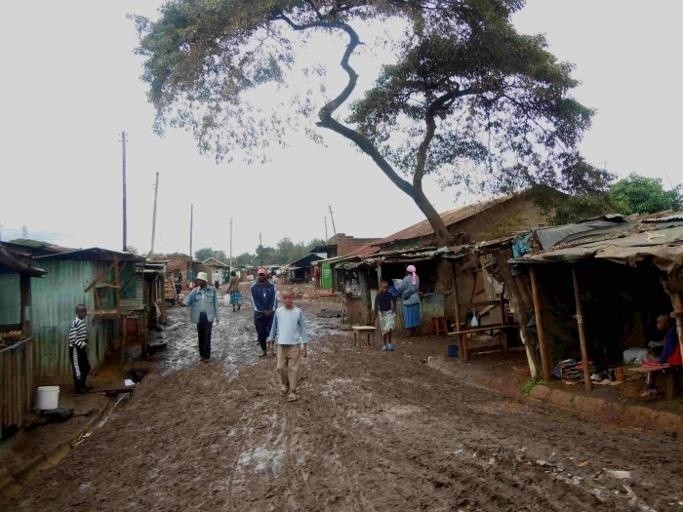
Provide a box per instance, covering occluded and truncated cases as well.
[278,385,297,402]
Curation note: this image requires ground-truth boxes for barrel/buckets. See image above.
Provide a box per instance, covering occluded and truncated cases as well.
[223,292,230,306]
[35,385,60,410]
[448,343,458,357]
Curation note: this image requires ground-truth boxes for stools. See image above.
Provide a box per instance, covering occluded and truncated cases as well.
[430,316,448,336]
[352,326,377,349]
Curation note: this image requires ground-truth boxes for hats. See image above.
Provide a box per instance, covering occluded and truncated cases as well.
[196,270,208,282]
[257,268,265,275]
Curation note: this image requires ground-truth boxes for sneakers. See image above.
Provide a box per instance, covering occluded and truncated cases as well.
[381,343,396,351]
[198,357,211,363]
[256,351,280,358]
[73,385,92,394]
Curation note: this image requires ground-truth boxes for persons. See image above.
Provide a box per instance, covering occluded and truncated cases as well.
[651,315,682,398]
[266,288,310,402]
[372,280,398,351]
[396,264,421,337]
[177,271,220,363]
[212,269,221,288]
[312,268,320,290]
[249,268,278,358]
[65,302,92,393]
[225,271,242,312]
